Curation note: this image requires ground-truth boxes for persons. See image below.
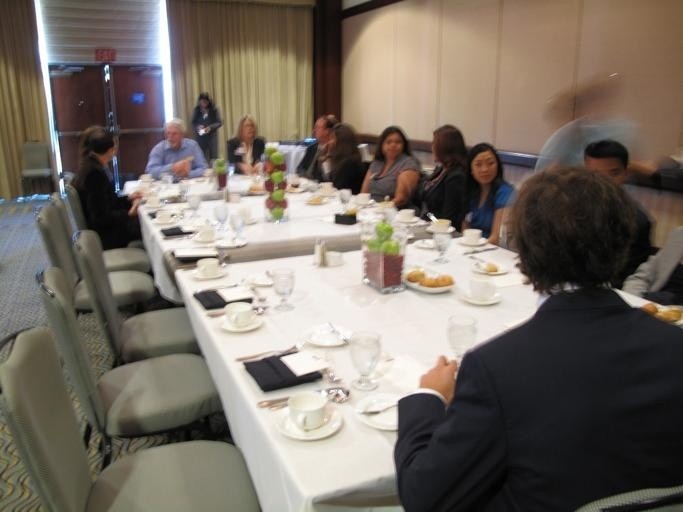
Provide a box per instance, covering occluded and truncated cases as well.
[393,165,680,511]
[500,68,662,251]
[359,125,420,210]
[226,115,265,176]
[582,139,655,297]
[619,222,683,305]
[190,92,222,162]
[144,119,210,184]
[74,126,147,249]
[296,114,365,195]
[455,141,517,245]
[411,124,469,235]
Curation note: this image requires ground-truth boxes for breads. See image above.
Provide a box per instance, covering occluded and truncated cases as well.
[409,269,451,289]
[485,261,499,273]
[642,303,682,324]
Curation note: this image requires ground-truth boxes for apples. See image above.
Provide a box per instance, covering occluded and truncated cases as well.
[261,147,288,220]
[364,221,401,254]
[214,157,228,175]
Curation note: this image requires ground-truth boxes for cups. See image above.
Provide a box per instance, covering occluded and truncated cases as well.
[136,167,514,443]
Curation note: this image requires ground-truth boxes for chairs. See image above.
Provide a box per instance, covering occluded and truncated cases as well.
[21,139,53,198]
